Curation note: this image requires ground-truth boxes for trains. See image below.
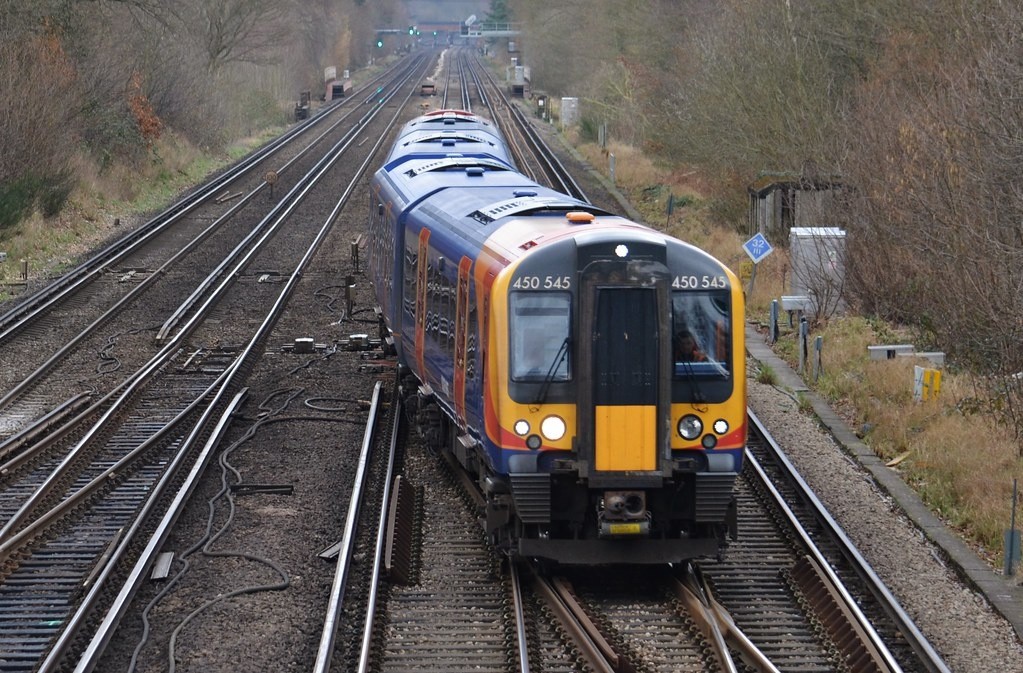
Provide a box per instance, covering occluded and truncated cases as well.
[369,108,744,564]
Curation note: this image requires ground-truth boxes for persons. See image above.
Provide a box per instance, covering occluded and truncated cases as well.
[677,331,708,362]
